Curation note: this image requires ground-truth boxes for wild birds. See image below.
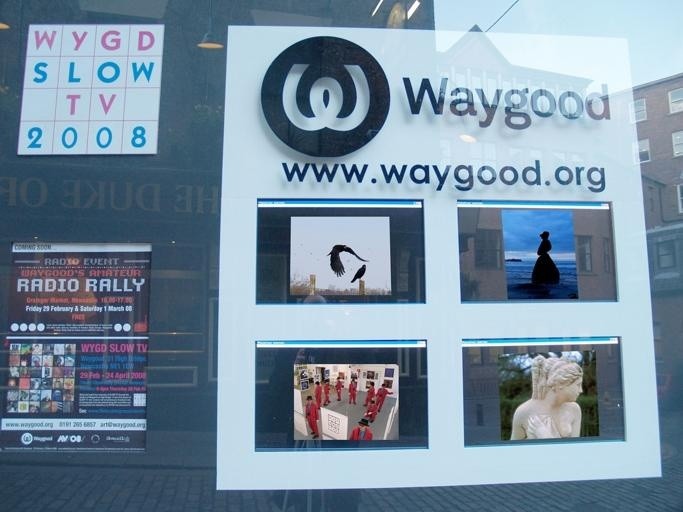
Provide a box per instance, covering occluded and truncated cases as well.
[327,245,368,278]
[351,265,366,283]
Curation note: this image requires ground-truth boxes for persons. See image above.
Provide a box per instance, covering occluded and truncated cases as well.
[531,231,559,284]
[361,381,375,406]
[362,399,378,423]
[509,354,583,440]
[347,379,357,405]
[323,379,331,406]
[313,380,322,409]
[334,376,342,401]
[348,418,372,440]
[375,383,393,413]
[303,395,320,439]
